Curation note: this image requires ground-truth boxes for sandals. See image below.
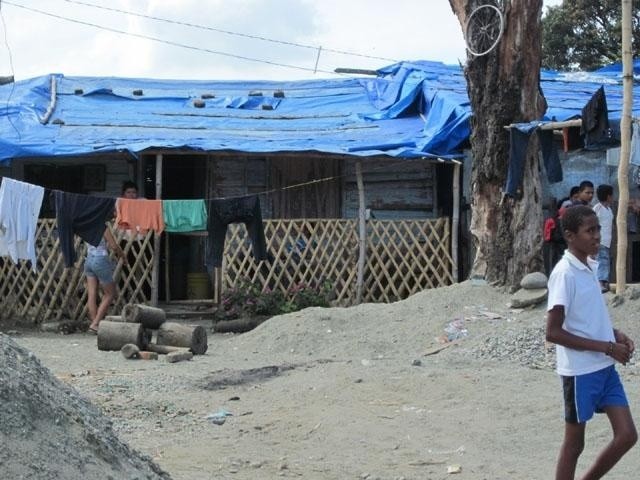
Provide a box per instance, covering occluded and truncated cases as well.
[86,327,98,336]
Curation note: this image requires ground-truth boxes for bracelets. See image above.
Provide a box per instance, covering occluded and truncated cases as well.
[605,341,614,355]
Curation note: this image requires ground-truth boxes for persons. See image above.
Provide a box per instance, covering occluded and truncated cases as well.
[551,180,615,293]
[543,202,637,480]
[83,225,129,335]
[120,180,149,297]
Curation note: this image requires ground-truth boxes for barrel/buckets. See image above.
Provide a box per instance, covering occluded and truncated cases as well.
[185,272,209,299]
